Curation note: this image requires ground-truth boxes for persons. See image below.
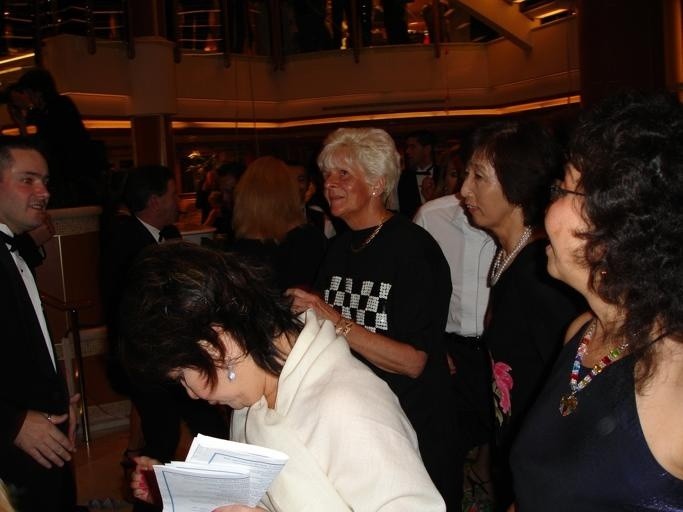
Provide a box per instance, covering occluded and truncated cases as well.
[1,64,683,510]
[170,0,454,55]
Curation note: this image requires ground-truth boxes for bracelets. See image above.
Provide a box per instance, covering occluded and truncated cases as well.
[339,321,352,336]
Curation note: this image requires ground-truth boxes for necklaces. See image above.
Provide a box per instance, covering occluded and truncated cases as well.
[556,338,622,414]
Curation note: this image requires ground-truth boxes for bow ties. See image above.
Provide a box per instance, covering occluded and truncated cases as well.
[415,166,434,176]
[0,231,25,253]
[157,230,170,242]
